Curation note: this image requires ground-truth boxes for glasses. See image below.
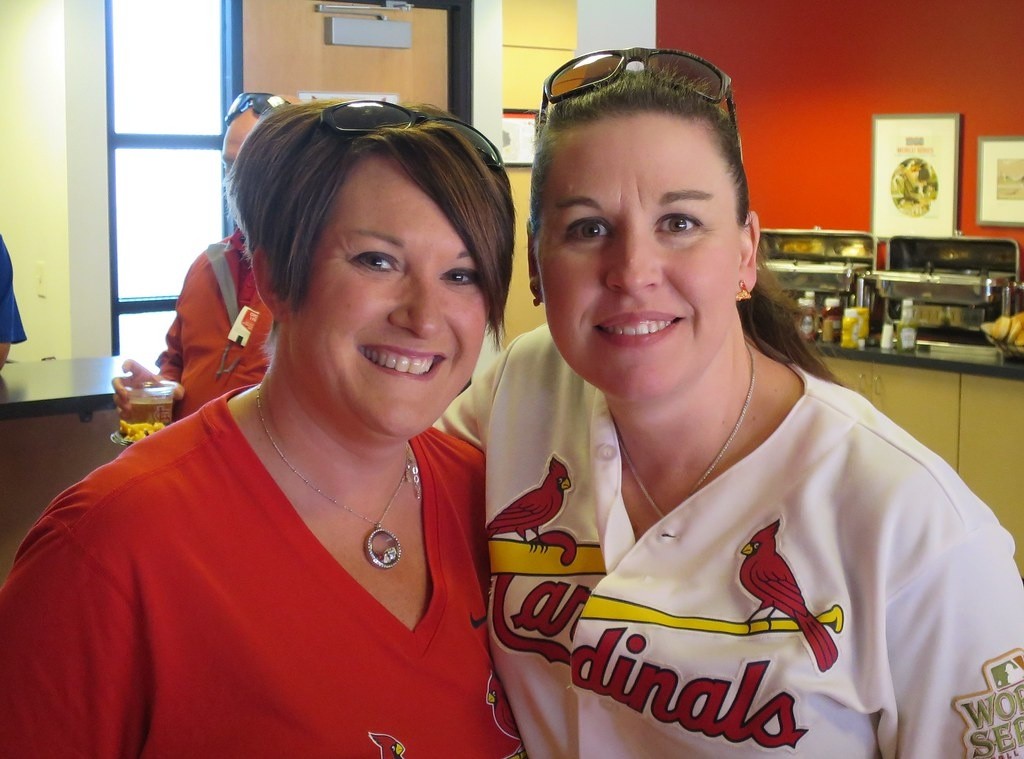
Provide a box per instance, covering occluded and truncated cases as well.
[319,100,503,173]
[540,47,743,164]
[224,92,291,126]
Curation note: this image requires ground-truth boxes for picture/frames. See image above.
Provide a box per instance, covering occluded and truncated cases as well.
[868,112,963,239]
[974,133,1024,229]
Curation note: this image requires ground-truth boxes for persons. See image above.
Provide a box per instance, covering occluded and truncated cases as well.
[105,104,282,435]
[0,234,25,371]
[431,68,1024,758]
[0,100,537,759]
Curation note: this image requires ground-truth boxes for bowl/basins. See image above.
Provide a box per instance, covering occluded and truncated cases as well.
[980,322,1024,358]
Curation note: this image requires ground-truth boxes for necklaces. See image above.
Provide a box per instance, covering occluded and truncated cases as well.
[252,387,425,576]
[617,336,759,525]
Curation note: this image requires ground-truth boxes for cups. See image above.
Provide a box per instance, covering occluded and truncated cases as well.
[118,381,173,441]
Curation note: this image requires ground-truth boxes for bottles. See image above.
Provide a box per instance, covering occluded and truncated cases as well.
[897,300,917,353]
[841,308,858,349]
[881,323,894,349]
